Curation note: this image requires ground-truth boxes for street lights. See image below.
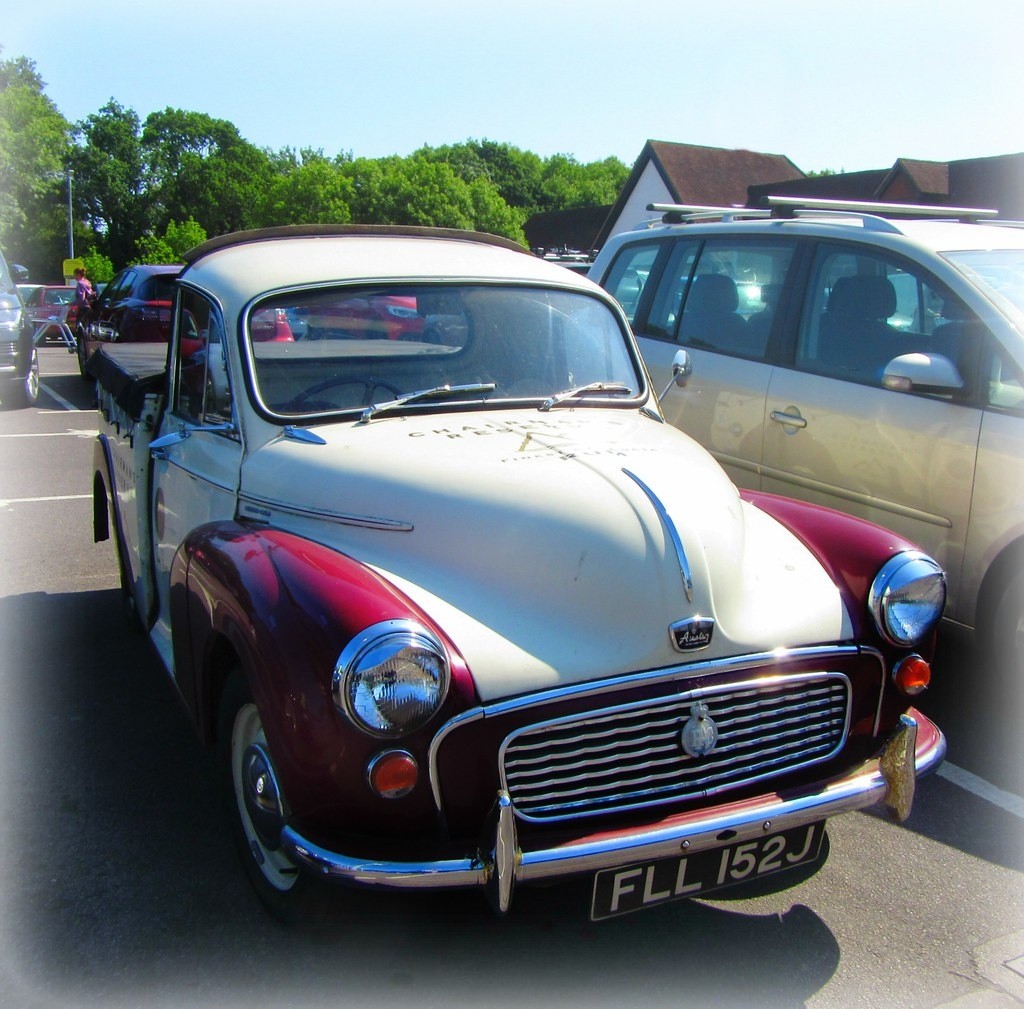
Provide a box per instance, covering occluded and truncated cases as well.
[65,168,76,258]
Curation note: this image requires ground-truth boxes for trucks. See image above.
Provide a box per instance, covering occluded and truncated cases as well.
[87,224,953,923]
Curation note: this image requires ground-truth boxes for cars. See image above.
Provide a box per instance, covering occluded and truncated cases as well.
[573,191,1024,715]
[67,262,294,398]
[16,281,110,345]
[557,255,947,333]
[284,295,466,349]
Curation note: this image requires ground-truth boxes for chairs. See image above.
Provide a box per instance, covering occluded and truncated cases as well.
[813,273,907,388]
[926,300,980,380]
[745,284,783,358]
[672,275,754,352]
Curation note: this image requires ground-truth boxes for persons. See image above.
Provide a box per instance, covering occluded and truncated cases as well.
[70,267,92,353]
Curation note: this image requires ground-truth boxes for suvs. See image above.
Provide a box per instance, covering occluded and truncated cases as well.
[0,250,41,407]
